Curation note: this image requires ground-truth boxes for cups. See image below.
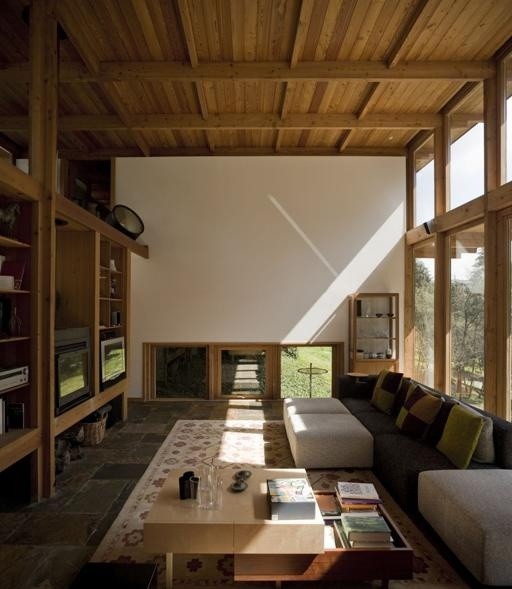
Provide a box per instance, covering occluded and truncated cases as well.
[111,310,121,325]
[1,275,15,289]
[178,466,224,510]
[231,471,252,490]
[376,313,394,317]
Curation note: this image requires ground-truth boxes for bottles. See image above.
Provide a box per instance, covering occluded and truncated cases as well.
[357,349,392,360]
[111,259,116,271]
[0,255,6,274]
[7,307,23,336]
[111,279,120,298]
[364,301,374,318]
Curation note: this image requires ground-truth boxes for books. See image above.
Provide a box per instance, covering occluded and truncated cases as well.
[265,476,396,550]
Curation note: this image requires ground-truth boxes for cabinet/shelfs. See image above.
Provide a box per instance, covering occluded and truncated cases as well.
[346,292,400,375]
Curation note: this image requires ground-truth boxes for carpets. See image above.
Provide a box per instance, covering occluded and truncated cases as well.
[88,418,470,588]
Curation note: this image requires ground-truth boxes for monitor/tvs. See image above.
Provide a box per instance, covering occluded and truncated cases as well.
[100,336,126,383]
[56,346,92,407]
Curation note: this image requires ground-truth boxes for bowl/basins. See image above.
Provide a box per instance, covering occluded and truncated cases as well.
[106,204,146,242]
